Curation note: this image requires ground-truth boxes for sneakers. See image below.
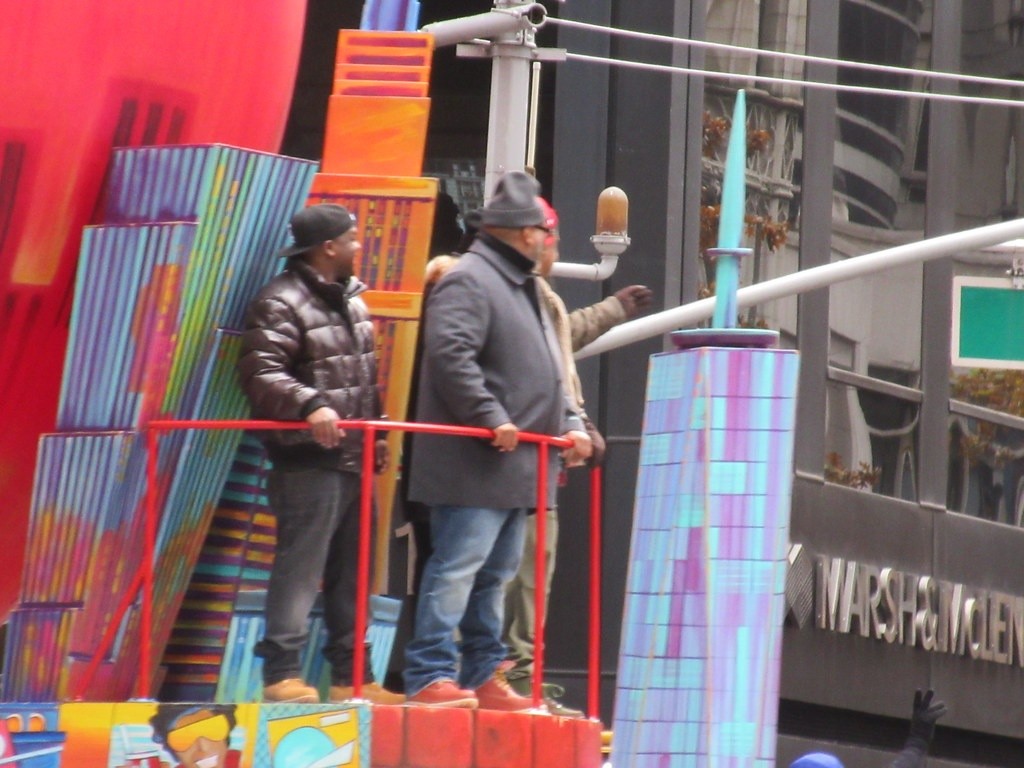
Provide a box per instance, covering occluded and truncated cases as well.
[476,660,534,712]
[327,681,407,705]
[402,679,479,710]
[263,678,320,703]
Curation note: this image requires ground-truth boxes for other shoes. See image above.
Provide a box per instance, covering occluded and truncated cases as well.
[543,696,584,715]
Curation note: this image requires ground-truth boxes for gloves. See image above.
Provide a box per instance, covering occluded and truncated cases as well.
[614,282,655,318]
[585,423,607,470]
[906,689,947,750]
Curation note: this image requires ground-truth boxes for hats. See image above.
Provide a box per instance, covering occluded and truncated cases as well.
[482,171,547,226]
[535,195,559,229]
[278,203,353,257]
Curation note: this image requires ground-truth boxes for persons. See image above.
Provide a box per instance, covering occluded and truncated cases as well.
[791,688,948,768]
[399,170,654,712]
[236,204,408,703]
